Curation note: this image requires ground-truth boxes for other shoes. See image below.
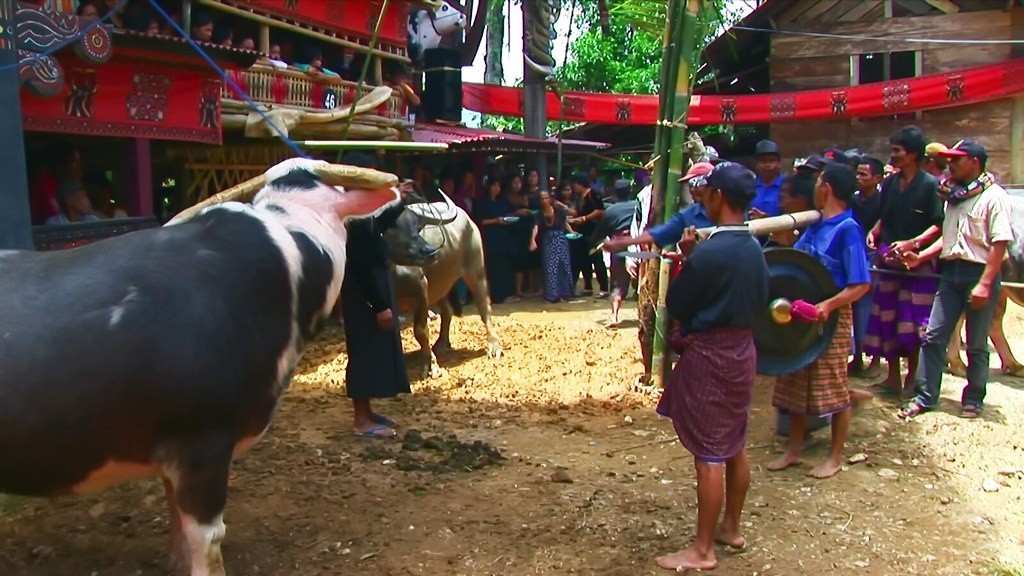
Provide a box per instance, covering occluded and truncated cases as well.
[595,292,610,299]
[575,290,593,297]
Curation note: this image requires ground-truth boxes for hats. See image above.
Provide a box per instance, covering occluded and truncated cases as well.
[688,161,757,207]
[756,140,779,155]
[678,162,715,183]
[817,148,850,167]
[924,142,951,164]
[796,155,824,172]
[937,139,988,162]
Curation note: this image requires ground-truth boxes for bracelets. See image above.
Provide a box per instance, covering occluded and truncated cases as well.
[911,238,921,251]
[583,216,586,223]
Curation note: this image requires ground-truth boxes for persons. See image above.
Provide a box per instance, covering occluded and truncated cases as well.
[29,140,132,226]
[342,150,415,436]
[655,160,774,573]
[437,161,712,390]
[79,4,420,108]
[749,123,1024,481]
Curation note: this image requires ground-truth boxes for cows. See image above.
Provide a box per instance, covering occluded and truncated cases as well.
[383,186,502,379]
[405,0,467,65]
[0,157,399,576]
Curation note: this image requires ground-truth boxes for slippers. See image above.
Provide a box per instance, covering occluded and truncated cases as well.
[960,403,981,417]
[864,383,901,395]
[897,398,928,418]
[353,425,396,438]
[377,416,397,428]
[899,390,916,401]
